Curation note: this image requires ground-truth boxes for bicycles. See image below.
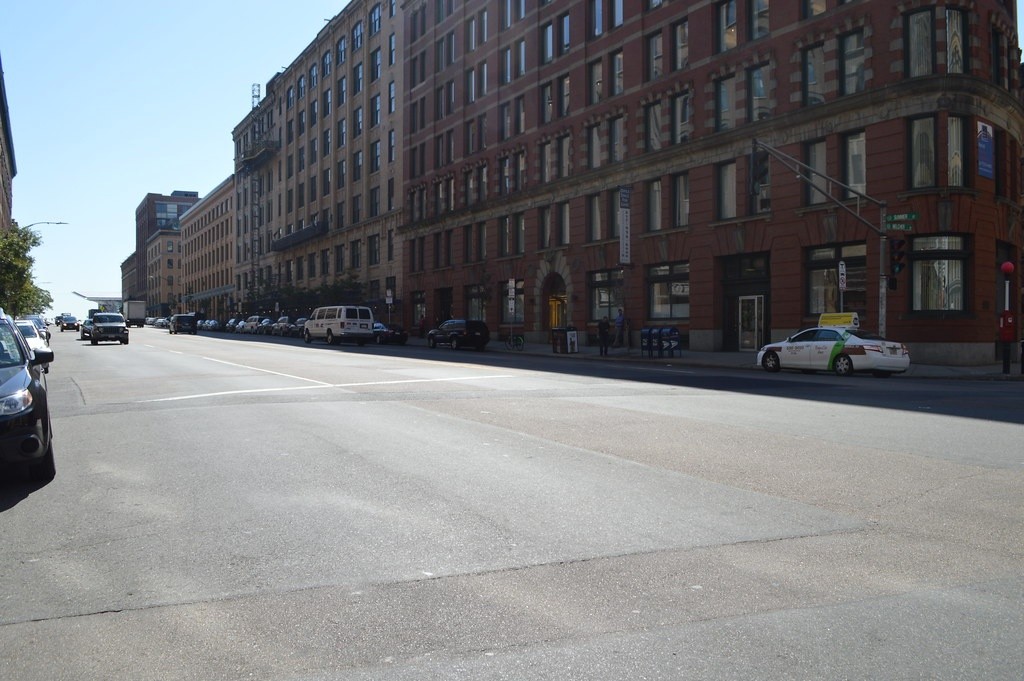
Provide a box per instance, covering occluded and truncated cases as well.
[505,331,525,351]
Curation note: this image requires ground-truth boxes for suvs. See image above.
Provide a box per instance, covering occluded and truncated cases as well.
[0,308,54,465]
[90,315,129,345]
[427,320,490,352]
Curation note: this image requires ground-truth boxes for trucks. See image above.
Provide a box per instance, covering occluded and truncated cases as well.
[123,301,146,328]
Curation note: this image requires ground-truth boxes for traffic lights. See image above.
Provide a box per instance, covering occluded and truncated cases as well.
[889,240,905,290]
[750,151,769,197]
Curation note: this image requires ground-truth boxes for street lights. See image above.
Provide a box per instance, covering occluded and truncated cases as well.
[150,276,173,317]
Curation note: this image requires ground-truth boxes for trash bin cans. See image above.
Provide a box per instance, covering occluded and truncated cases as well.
[661,327,681,358]
[650,328,661,358]
[552,327,578,354]
[641,328,651,358]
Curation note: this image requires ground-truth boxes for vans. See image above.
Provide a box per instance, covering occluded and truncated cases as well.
[303,306,374,346]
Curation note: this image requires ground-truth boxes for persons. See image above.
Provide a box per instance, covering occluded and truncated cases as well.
[598,316,610,356]
[419,315,424,338]
[613,309,623,346]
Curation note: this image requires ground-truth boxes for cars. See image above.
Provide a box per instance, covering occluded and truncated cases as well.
[374,322,409,345]
[14,315,51,374]
[197,315,307,338]
[756,312,911,378]
[54,312,93,340]
[145,312,197,335]
